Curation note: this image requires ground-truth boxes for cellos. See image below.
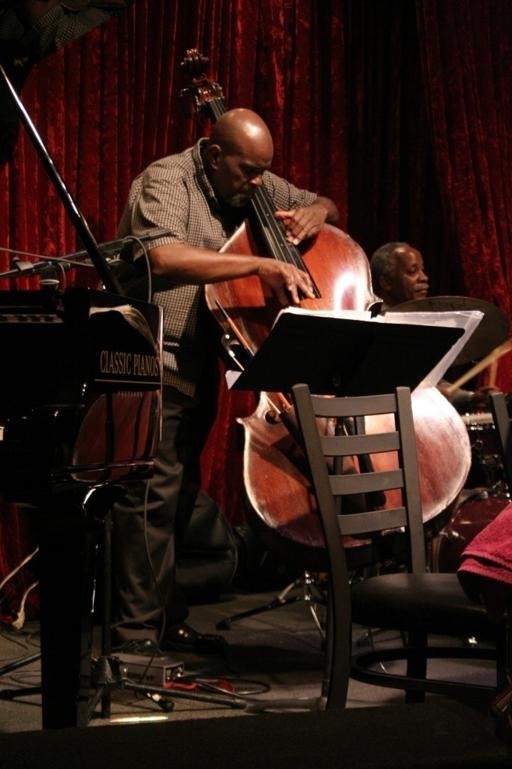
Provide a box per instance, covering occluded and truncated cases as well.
[176,48,472,552]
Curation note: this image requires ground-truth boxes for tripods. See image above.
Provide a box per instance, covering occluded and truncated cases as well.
[0,64,174,725]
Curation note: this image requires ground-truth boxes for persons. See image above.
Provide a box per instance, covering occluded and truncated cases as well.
[109,109,342,656]
[370,242,501,414]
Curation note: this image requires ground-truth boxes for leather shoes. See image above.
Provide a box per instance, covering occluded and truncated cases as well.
[172,625,229,650]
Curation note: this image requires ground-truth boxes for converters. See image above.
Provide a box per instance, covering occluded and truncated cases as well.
[110,651,184,688]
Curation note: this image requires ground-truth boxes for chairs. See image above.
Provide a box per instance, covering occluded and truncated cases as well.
[284,380,512,711]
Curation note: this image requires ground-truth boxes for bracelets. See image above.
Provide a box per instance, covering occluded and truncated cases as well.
[466,390,475,408]
